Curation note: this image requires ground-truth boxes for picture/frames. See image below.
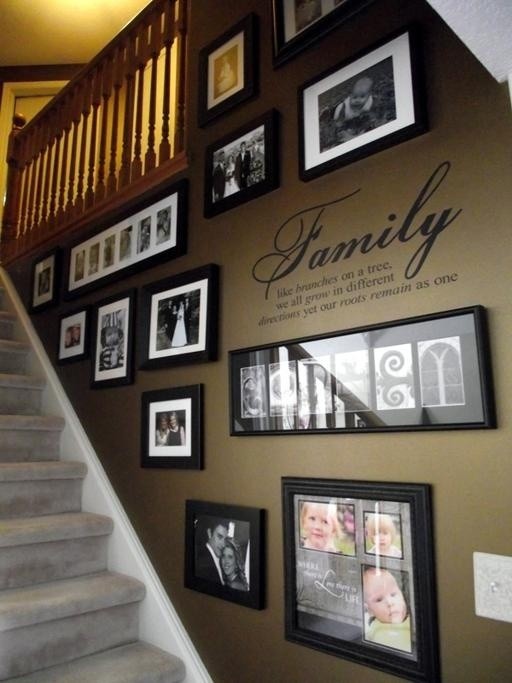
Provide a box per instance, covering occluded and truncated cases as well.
[281,475,442,683]
[90,287,136,390]
[63,178,188,303]
[140,383,203,470]
[204,109,281,219]
[136,262,219,371]
[271,0,375,71]
[57,304,90,364]
[228,305,497,437]
[198,13,260,129]
[297,26,427,183]
[29,245,63,314]
[183,499,266,611]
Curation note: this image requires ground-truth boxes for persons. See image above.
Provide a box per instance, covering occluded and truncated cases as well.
[211,151,226,201]
[71,325,81,346]
[334,76,386,143]
[366,514,403,558]
[164,296,190,346]
[301,502,341,556]
[219,542,249,592]
[165,411,186,445]
[361,566,413,656]
[155,413,167,445]
[98,313,125,369]
[235,142,251,190]
[64,328,73,347]
[196,518,229,584]
